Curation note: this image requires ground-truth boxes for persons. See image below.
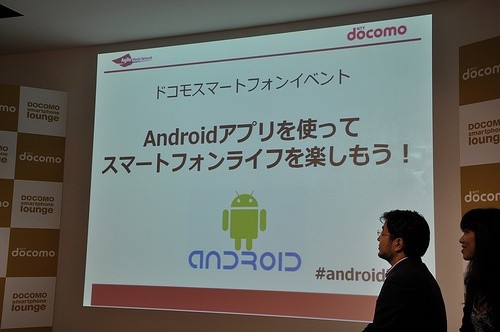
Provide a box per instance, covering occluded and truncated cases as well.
[458,207,500,332]
[361,209,448,332]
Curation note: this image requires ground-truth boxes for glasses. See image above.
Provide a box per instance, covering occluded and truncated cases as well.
[377,230,393,237]
